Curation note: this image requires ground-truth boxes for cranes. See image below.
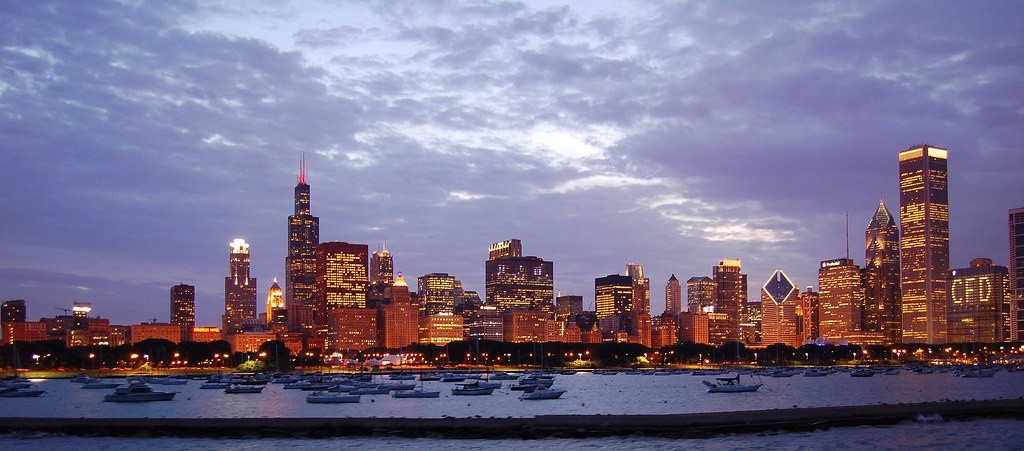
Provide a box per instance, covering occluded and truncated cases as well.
[56,308,70,315]
[148,315,157,322]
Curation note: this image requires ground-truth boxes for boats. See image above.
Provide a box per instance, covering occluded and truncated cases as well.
[71,372,189,403]
[201,335,577,401]
[0,376,45,397]
[703,336,763,395]
[593,364,1024,379]
